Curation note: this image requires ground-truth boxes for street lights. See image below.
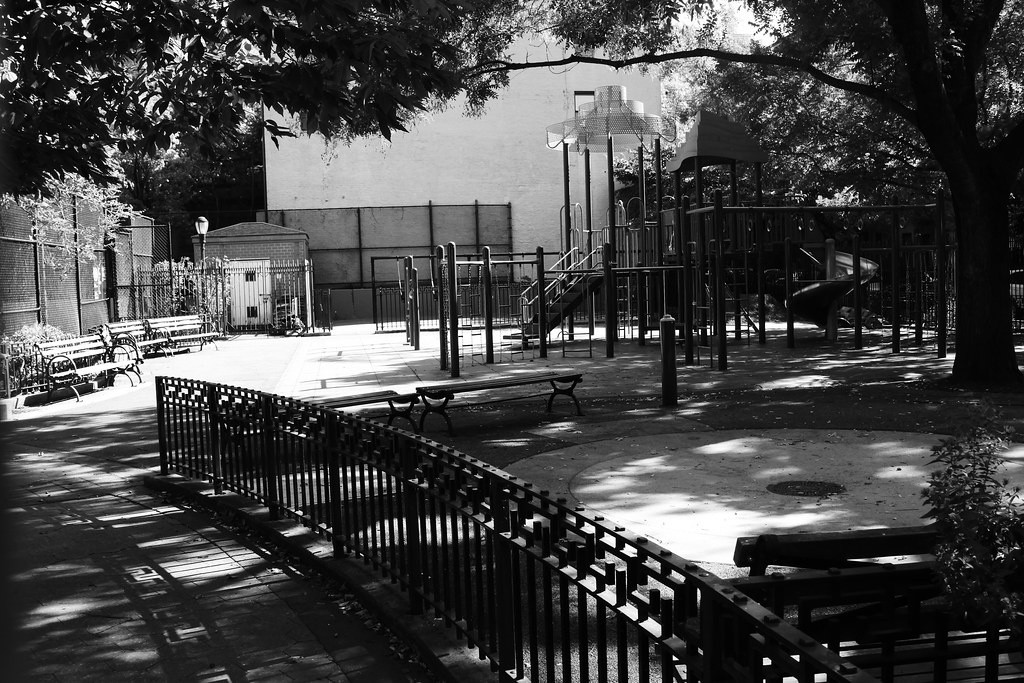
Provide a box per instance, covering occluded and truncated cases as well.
[194,216,209,334]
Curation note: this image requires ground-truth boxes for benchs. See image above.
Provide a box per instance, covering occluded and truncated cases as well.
[297,390,421,434]
[720,514,1024,683]
[89,326,145,384]
[416,368,583,433]
[34,333,136,402]
[145,313,220,357]
[95,318,168,365]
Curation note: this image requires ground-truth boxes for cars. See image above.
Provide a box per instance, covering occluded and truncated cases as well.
[769,251,1024,331]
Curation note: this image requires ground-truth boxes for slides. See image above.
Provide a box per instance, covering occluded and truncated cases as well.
[787,249,882,328]
